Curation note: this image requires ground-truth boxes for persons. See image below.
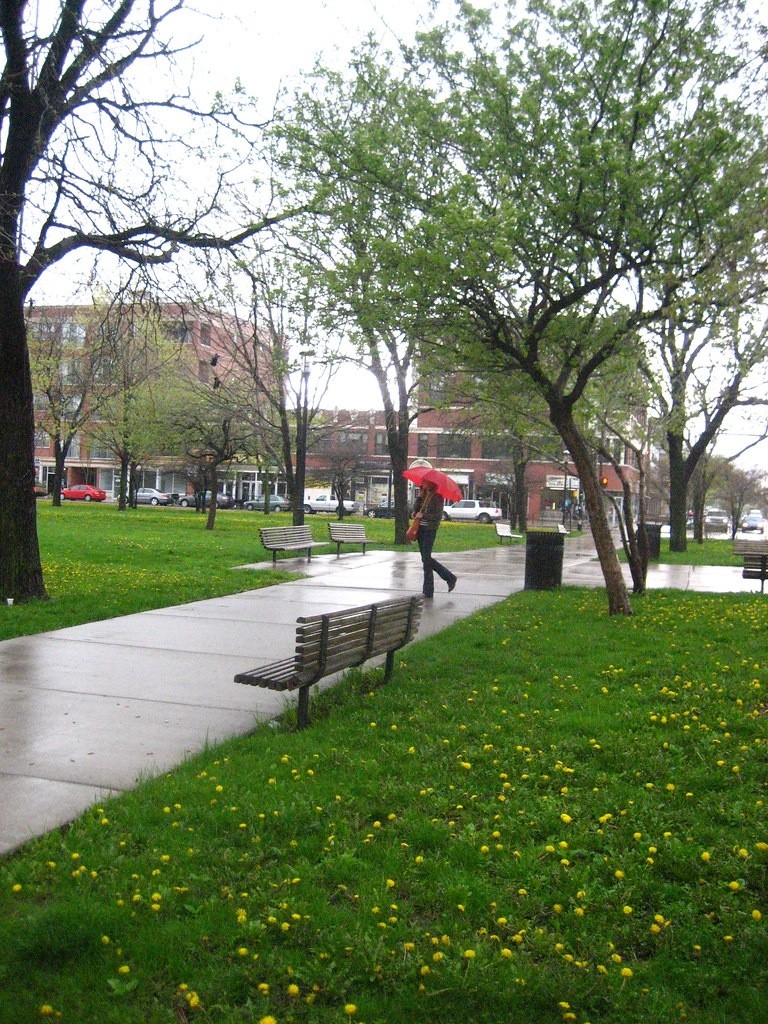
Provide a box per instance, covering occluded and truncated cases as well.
[406,478,457,599]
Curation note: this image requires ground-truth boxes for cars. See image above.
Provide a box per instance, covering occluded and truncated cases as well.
[58,484,107,502]
[117,487,174,506]
[704,509,730,534]
[242,494,291,513]
[33,481,47,496]
[739,509,764,535]
[364,501,415,519]
[177,490,236,509]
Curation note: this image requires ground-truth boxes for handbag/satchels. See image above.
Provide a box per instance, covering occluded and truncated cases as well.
[405,518,420,541]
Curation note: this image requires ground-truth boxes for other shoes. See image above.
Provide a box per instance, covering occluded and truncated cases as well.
[423,592,433,598]
[447,575,457,592]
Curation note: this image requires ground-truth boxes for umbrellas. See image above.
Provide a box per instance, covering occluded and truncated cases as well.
[402,465,462,522]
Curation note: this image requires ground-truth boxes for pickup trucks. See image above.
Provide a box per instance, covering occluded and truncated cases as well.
[440,500,503,524]
[304,494,357,516]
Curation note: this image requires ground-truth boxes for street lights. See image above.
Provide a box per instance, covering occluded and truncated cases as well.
[562,449,571,527]
[299,365,312,496]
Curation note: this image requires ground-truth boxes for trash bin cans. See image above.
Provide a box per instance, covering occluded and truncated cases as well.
[638,523,664,560]
[524,530,567,587]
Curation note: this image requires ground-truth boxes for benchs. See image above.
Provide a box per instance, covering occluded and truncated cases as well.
[742,555,768,595]
[495,523,523,544]
[557,525,570,536]
[235,592,424,730]
[732,539,768,556]
[257,524,330,563]
[327,522,373,560]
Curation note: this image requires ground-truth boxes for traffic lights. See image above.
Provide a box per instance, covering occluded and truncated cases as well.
[599,477,609,489]
